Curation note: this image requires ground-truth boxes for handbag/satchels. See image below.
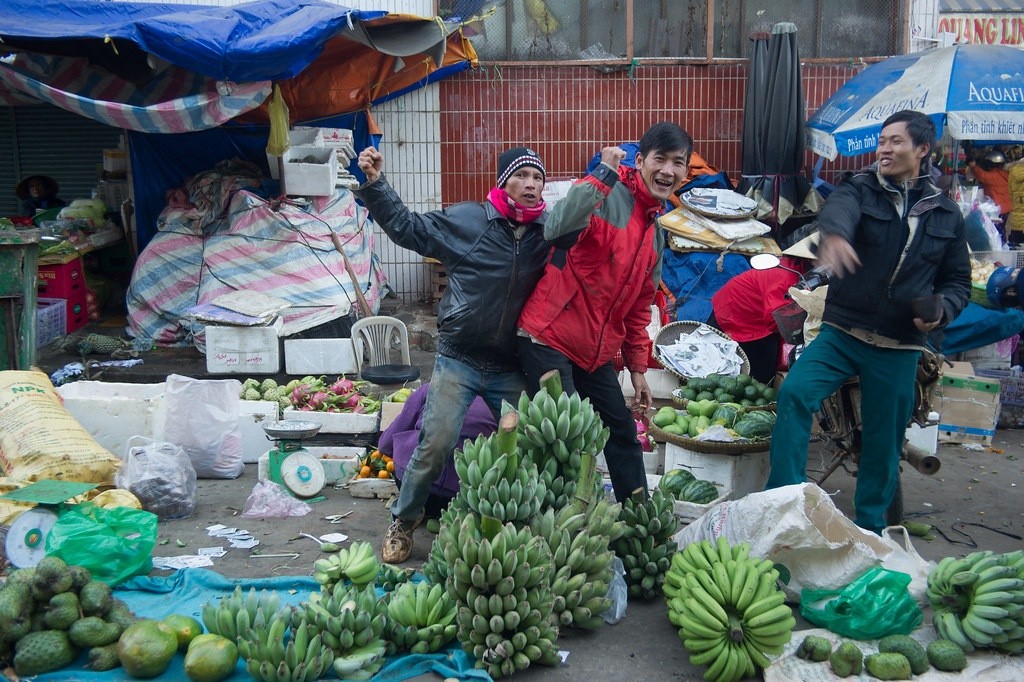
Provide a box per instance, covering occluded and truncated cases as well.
[117,435,199,521]
[44,505,158,590]
[158,373,246,481]
[799,564,923,641]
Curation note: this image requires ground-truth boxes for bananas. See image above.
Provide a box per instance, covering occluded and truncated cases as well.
[201,541,391,682]
[662,535,796,682]
[378,386,679,678]
[927,549,1024,656]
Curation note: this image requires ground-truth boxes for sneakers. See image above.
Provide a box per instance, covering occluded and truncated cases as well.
[382,505,425,564]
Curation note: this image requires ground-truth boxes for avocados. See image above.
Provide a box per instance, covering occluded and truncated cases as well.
[681,373,777,407]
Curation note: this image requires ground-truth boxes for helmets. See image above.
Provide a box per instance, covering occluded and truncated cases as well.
[984,150,1005,167]
[986,266,1022,310]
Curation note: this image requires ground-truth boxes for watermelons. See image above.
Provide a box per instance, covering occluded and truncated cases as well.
[657,469,719,504]
[711,405,776,440]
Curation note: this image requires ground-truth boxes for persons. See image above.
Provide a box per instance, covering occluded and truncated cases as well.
[377,382,497,521]
[353,148,554,563]
[766,111,973,537]
[965,152,1024,246]
[16,172,67,220]
[516,122,693,502]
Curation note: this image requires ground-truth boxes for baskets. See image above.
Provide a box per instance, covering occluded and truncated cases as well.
[973,250,1024,268]
[772,301,805,345]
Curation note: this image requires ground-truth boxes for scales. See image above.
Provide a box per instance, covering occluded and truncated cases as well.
[262,420,326,505]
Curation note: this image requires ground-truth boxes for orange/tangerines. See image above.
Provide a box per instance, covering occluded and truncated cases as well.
[355,450,395,480]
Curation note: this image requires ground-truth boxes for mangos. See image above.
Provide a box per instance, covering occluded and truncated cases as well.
[653,399,718,438]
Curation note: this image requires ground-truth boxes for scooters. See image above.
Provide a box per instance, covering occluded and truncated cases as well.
[749,252,954,530]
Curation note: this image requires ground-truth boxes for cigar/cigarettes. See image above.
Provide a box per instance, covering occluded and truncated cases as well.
[640,404,657,410]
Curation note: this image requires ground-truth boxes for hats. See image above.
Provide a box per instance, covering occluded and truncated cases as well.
[15,173,60,200]
[497,146,545,191]
[782,230,822,260]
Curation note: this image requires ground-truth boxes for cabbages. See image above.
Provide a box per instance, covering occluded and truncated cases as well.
[58,198,107,230]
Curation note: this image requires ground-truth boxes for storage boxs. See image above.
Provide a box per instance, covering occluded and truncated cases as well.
[238,401,279,463]
[206,314,283,373]
[971,244,1024,308]
[266,126,355,196]
[55,381,167,460]
[665,441,770,501]
[37,298,67,349]
[379,389,417,431]
[611,290,679,399]
[284,338,358,374]
[937,362,1001,447]
[595,448,659,474]
[284,405,378,433]
[89,148,129,247]
[259,447,367,486]
[646,474,734,524]
[959,338,1012,369]
[974,368,1024,407]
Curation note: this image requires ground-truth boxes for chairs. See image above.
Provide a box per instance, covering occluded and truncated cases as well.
[351,316,421,401]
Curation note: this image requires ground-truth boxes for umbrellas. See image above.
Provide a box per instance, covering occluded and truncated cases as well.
[737,20,810,240]
[801,41,1024,203]
